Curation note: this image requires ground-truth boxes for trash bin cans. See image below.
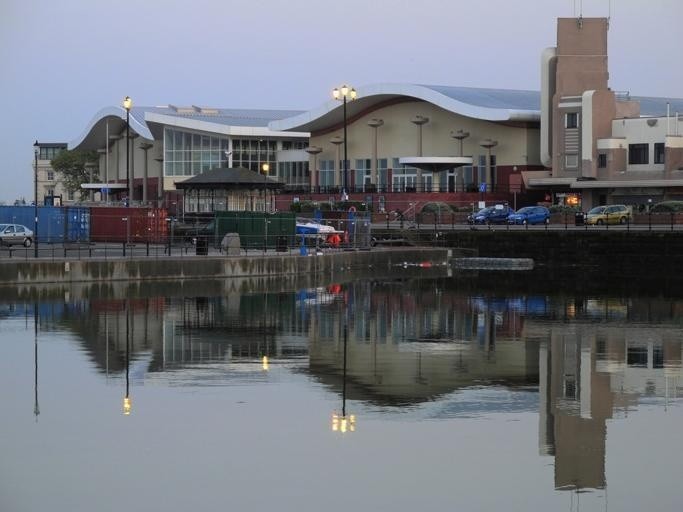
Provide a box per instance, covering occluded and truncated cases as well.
[276,236,287,252]
[575,212,584,226]
[196,237,208,255]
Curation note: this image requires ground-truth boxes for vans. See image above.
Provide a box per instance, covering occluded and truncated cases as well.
[584,205,632,226]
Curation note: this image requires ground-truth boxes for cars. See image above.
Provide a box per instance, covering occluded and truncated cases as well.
[1,223,34,247]
[184,220,215,245]
[466,206,551,226]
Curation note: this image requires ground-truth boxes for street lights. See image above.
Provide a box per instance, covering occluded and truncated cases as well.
[34,140,40,258]
[331,85,357,185]
[331,324,356,434]
[263,163,269,252]
[123,96,132,207]
[124,311,132,416]
[33,300,41,423]
[263,294,270,372]
